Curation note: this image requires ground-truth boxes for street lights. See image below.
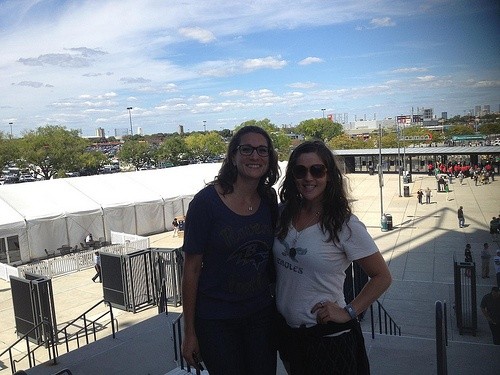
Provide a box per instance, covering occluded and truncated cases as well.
[321,109,326,118]
[8,122,13,140]
[126,106,133,136]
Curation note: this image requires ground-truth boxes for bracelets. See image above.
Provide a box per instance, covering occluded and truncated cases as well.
[344,305,356,321]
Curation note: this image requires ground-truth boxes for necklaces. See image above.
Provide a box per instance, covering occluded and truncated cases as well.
[291,204,322,257]
[231,187,255,212]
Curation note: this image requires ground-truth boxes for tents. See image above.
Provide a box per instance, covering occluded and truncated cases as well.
[1,161,347,264]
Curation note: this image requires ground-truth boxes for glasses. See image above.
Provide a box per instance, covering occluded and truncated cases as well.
[291,163,327,180]
[234,145,270,157]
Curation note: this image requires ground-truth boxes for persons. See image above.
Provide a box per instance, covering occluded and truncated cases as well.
[271,141,392,375]
[172,218,179,237]
[363,157,500,345]
[179,126,280,375]
[86,233,104,283]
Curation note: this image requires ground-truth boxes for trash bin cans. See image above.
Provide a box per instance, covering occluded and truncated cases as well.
[404,186,409,197]
[406,174,412,183]
[381,213,392,232]
[369,167,374,175]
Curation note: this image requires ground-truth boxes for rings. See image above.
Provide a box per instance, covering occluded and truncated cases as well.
[321,302,324,307]
